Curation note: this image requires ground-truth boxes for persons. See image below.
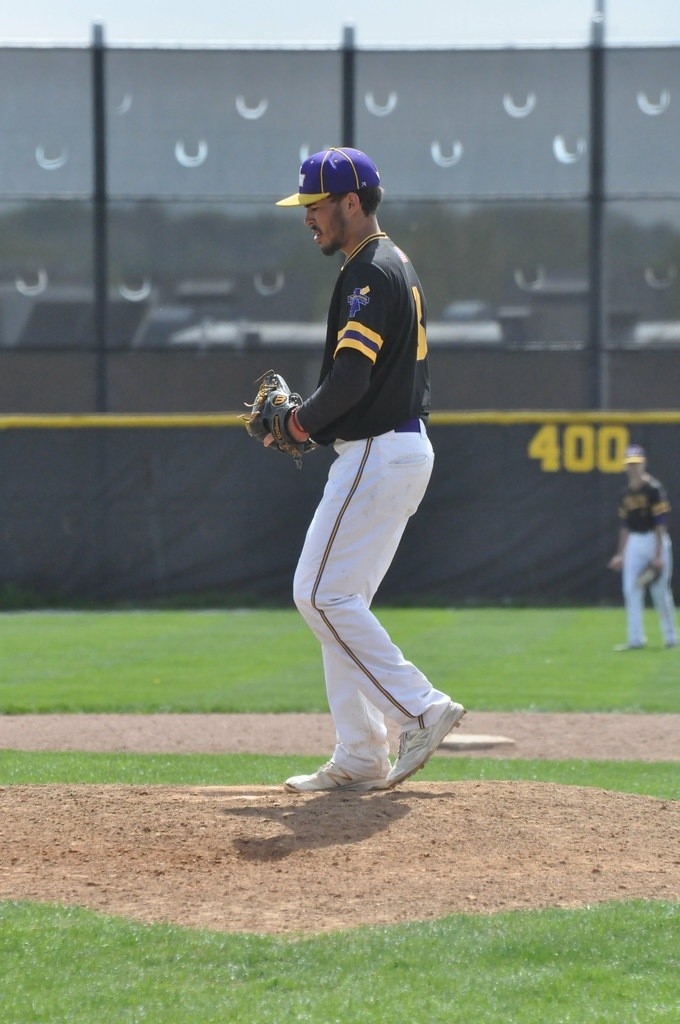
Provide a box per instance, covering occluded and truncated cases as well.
[608,446,680,652]
[245,147,469,792]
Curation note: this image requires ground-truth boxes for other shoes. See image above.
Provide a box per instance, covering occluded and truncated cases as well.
[613,641,647,651]
[664,640,674,648]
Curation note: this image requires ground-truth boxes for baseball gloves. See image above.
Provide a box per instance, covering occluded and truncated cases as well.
[636,562,663,589]
[245,368,315,459]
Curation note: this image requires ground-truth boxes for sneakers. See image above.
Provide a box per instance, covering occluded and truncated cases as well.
[284,743,393,793]
[385,700,467,786]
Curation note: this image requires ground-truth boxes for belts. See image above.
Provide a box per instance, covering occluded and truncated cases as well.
[394,417,421,432]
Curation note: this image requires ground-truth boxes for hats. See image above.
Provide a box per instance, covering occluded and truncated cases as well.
[274,148,381,207]
[623,444,648,465]
